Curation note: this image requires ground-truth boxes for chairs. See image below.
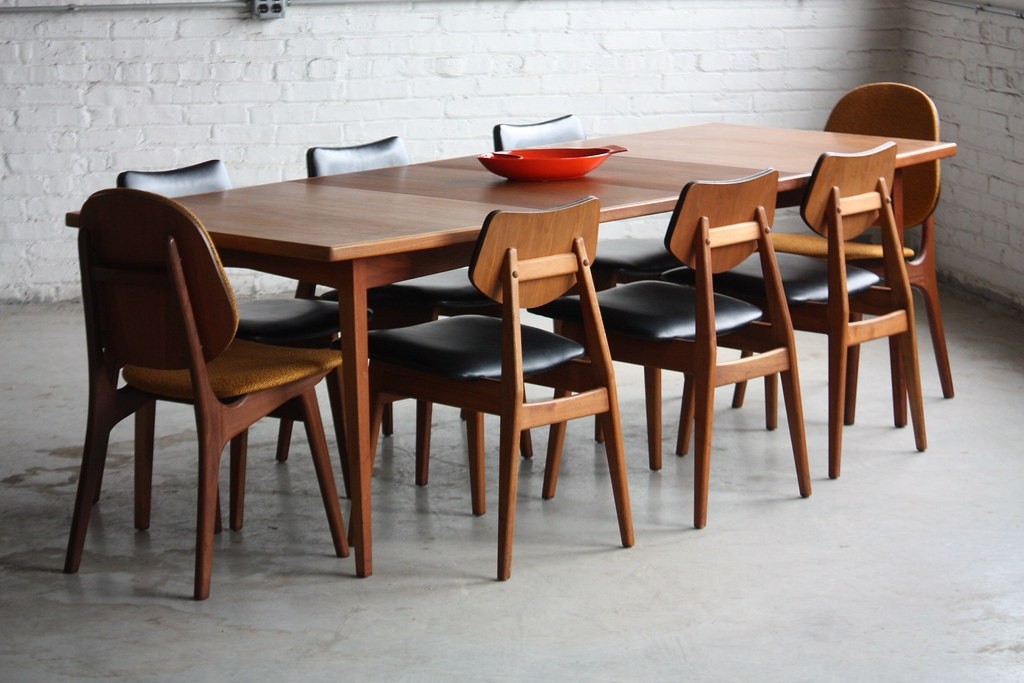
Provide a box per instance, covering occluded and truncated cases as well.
[63,83,956,600]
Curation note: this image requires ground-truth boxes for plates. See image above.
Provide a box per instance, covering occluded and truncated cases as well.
[477,143,627,181]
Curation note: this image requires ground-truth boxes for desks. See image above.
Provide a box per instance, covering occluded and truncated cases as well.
[133,125,955,579]
